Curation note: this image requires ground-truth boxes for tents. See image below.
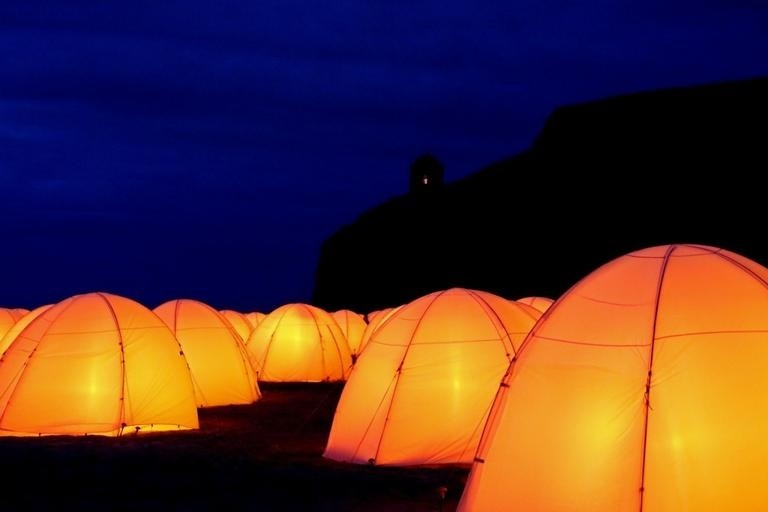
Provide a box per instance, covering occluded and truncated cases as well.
[1,293,267,438]
[246,286,555,466]
[452,238,768,511]
